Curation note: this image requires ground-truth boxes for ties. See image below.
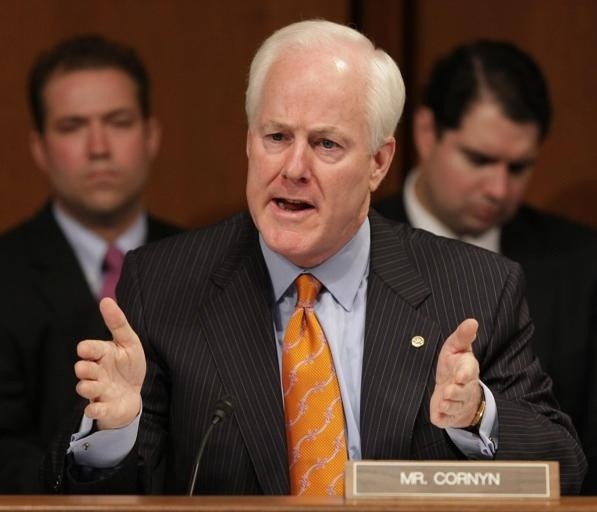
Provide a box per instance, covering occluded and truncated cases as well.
[281,270,350,499]
[100,244,127,298]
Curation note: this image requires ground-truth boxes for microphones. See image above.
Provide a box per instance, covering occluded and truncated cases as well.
[188,396,235,496]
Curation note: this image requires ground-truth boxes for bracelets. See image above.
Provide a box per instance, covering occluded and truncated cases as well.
[459,387,485,433]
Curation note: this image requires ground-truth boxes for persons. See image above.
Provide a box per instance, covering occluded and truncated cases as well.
[362,36,594,496]
[0,31,199,496]
[37,18,593,496]
